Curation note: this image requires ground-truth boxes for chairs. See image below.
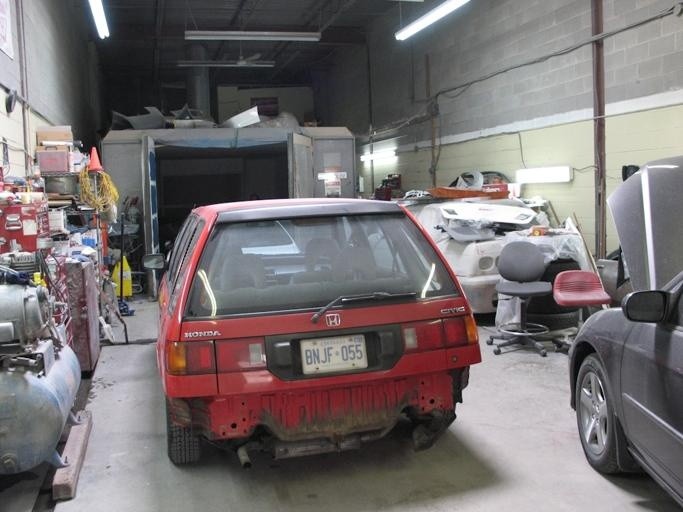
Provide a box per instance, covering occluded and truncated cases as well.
[485,239,561,358]
[209,239,411,313]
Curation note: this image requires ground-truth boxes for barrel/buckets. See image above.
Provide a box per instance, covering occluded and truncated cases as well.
[47,208,64,233]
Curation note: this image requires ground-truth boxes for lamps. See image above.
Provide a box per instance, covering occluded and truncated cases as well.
[184,0,321,42]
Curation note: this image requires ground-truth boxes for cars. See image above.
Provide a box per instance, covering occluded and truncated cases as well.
[568,154,683,510]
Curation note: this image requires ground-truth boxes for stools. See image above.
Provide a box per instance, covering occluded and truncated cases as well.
[552,270,612,354]
